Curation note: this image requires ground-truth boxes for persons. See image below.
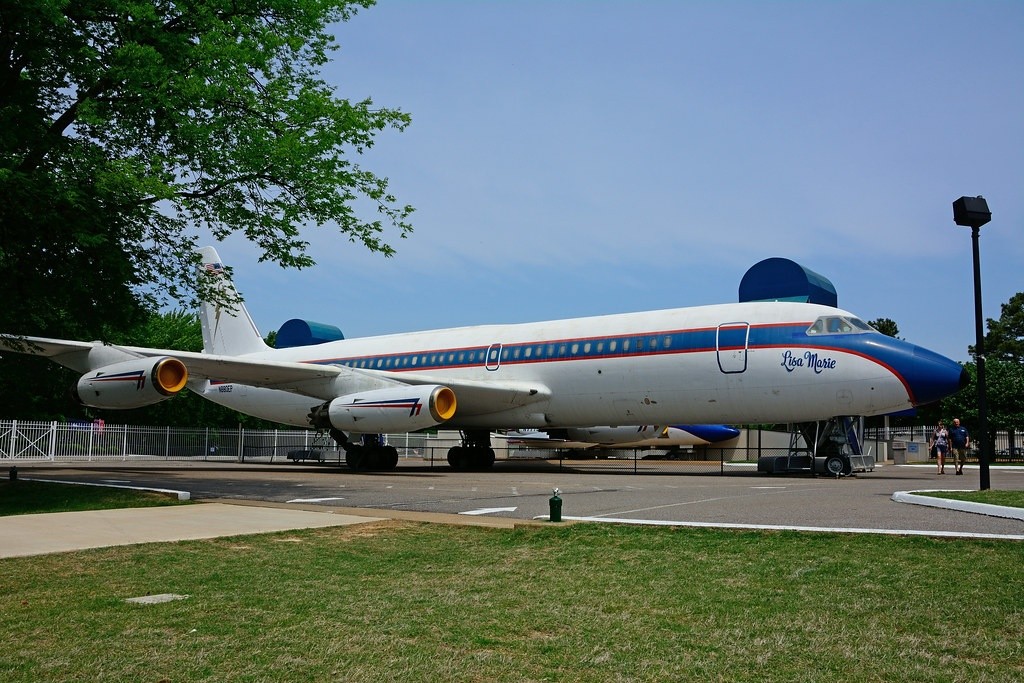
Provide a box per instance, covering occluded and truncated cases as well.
[948,418,969,475]
[928,421,950,476]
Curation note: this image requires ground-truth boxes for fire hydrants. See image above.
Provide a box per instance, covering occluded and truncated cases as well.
[548,488,564,522]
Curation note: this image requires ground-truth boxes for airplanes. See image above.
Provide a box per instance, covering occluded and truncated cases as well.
[0,246,976,474]
[496,422,741,457]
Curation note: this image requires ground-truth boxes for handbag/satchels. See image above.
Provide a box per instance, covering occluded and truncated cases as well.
[930,443,939,459]
